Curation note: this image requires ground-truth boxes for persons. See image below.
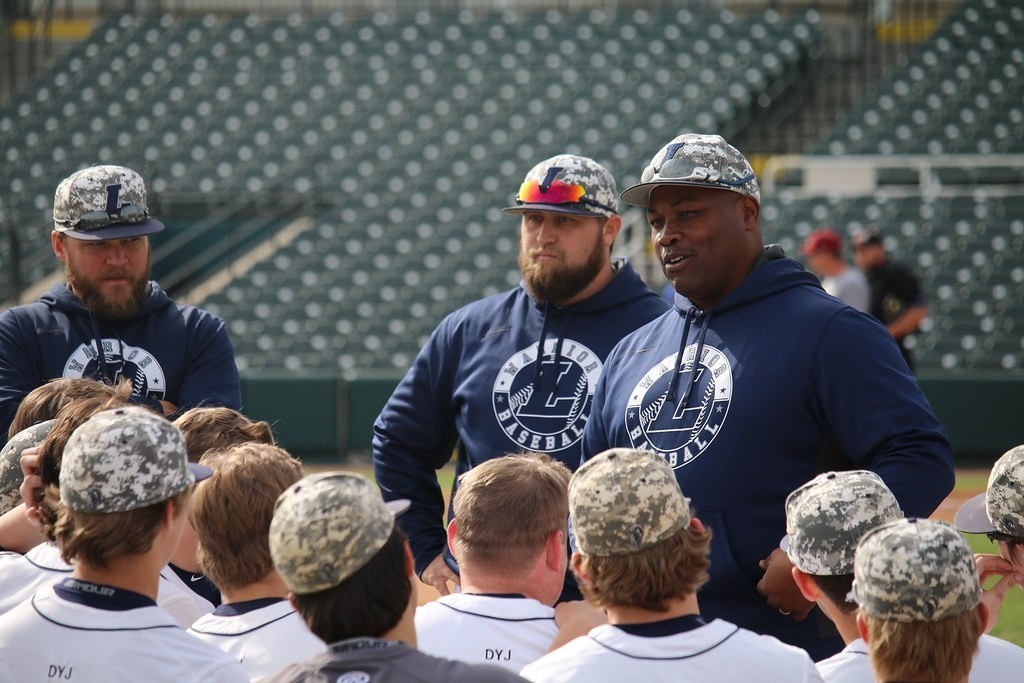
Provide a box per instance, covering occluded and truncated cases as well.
[0,164,242,451]
[566,133,956,661]
[410,451,573,674]
[803,230,873,319]
[0,377,328,683]
[845,516,988,683]
[258,471,534,683]
[954,444,1024,637]
[780,469,1024,683]
[370,153,670,600]
[852,227,928,372]
[517,446,827,683]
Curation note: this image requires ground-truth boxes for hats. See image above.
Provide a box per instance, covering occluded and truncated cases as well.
[845,516,984,624]
[620,133,762,217]
[54,163,163,240]
[569,446,693,558]
[59,406,212,513]
[952,445,1024,542]
[795,230,841,255]
[781,469,906,573]
[502,154,620,226]
[267,470,412,594]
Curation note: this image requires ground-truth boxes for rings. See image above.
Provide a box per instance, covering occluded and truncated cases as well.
[779,608,791,615]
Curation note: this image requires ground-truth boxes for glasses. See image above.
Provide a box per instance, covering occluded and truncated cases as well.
[638,160,754,188]
[514,179,619,219]
[54,204,150,232]
